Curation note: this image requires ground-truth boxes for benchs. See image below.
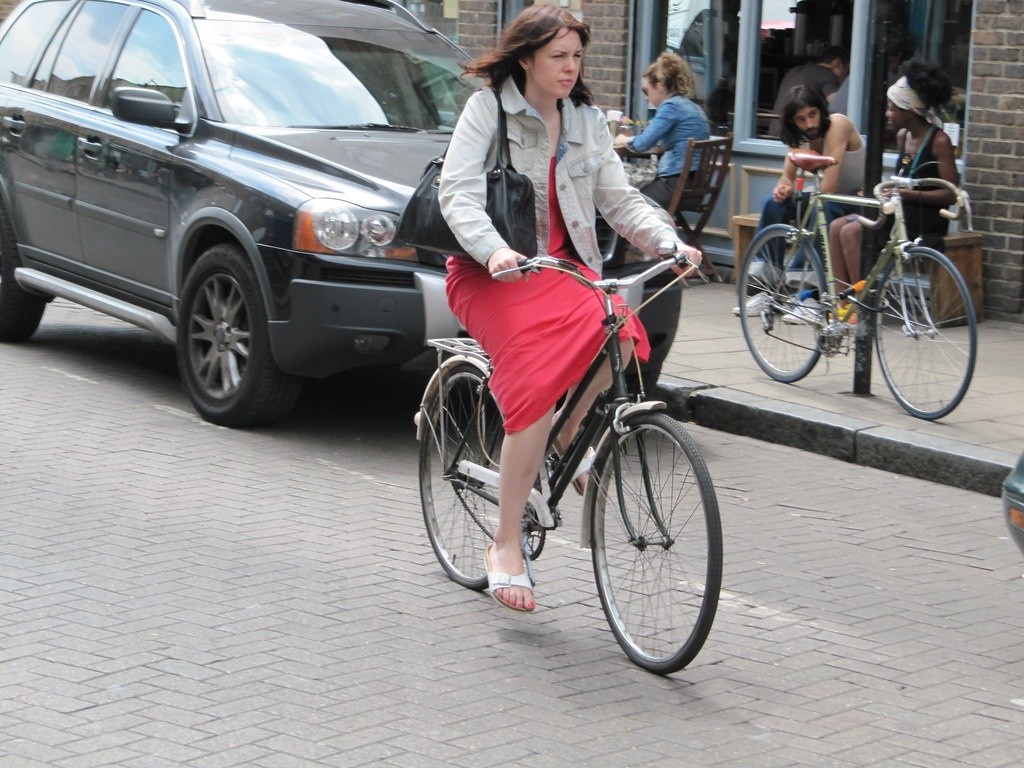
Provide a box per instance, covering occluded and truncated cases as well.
[732,213,983,329]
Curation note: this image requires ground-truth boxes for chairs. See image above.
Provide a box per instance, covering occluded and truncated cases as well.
[667,131,734,284]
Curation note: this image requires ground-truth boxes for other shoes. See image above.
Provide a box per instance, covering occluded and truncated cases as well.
[829,304,858,327]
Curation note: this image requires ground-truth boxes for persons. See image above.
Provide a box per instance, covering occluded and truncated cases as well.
[767,45,849,136]
[445,8,703,611]
[829,57,960,323]
[614,51,711,206]
[732,85,866,324]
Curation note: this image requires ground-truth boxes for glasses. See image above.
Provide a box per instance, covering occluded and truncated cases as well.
[643,80,657,96]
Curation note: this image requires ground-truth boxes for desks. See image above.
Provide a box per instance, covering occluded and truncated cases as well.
[612,138,664,158]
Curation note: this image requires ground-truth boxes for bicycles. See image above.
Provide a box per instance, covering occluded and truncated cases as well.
[413,240,724,676]
[737,150,978,421]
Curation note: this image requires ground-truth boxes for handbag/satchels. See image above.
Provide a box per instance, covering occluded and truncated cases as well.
[395,85,541,272]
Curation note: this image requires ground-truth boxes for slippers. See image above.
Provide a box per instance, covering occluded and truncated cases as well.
[550,418,596,496]
[483,544,534,612]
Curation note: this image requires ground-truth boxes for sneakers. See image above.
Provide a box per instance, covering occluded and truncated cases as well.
[732,290,774,317]
[781,297,822,325]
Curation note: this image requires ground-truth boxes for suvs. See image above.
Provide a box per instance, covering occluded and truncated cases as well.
[0,0,686,436]
